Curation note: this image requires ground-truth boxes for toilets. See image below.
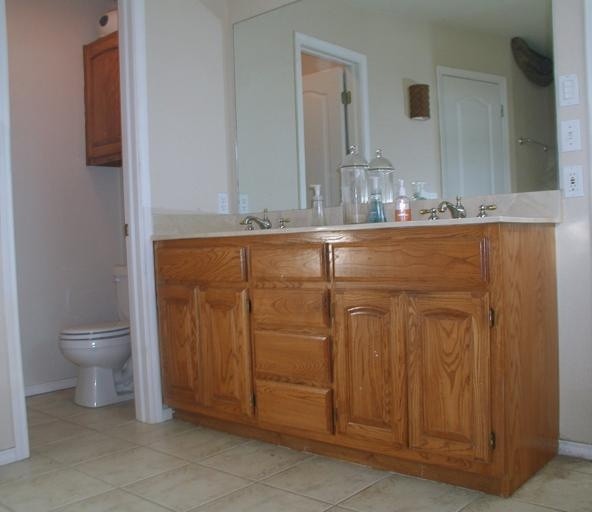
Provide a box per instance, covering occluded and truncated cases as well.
[59,264,131,407]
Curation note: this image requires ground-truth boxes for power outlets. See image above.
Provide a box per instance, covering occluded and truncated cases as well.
[217,192,230,215]
[238,193,249,214]
[561,121,586,199]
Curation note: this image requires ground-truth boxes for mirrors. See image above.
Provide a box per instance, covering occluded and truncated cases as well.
[231,1,560,215]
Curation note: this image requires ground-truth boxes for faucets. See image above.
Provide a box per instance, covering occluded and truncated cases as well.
[239,215,272,229]
[437,194,466,218]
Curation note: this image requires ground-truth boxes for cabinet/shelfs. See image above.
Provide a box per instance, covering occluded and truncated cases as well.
[83,30,123,168]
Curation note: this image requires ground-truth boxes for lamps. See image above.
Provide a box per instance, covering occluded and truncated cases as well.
[408,83,430,121]
